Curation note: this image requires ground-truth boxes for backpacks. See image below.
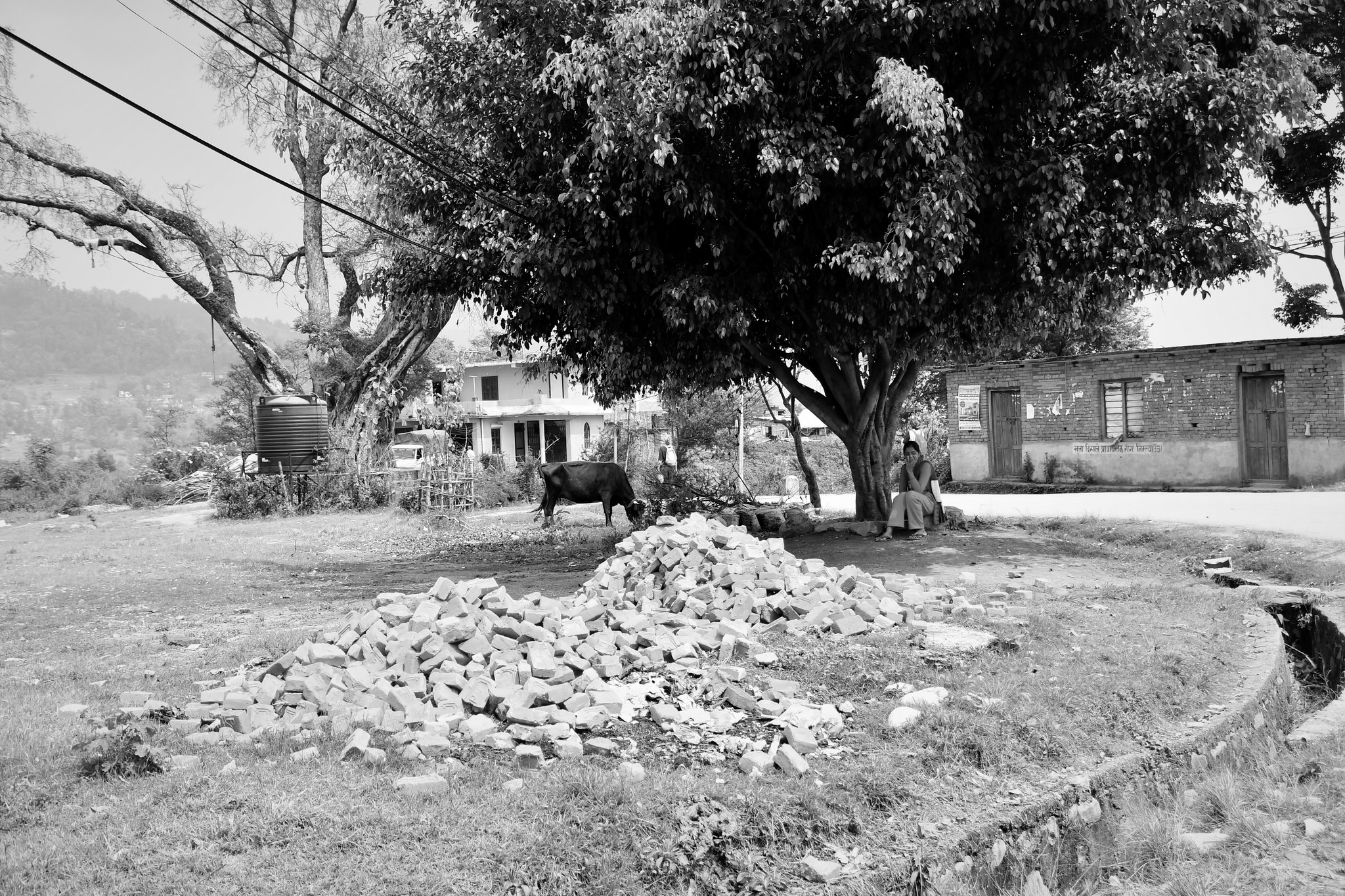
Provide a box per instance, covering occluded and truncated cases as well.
[911,429,927,455]
[663,445,677,466]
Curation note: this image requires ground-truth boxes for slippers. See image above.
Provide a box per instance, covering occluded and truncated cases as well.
[906,533,930,539]
[875,535,894,542]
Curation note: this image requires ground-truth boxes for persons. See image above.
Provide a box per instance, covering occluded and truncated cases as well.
[466,446,475,472]
[657,434,678,486]
[901,419,927,462]
[875,441,941,543]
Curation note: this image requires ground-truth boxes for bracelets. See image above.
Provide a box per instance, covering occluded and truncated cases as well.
[909,476,915,480]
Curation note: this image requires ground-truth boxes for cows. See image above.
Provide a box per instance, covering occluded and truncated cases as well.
[527,460,646,528]
